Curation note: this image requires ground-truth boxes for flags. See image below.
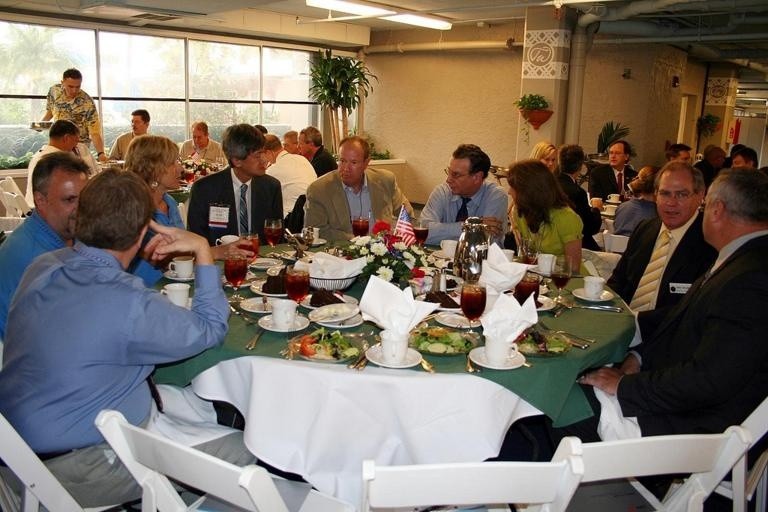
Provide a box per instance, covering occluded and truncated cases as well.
[393,203,416,247]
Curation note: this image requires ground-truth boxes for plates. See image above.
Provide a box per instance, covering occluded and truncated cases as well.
[316,314,364,329]
[310,238,328,246]
[469,346,527,371]
[309,304,360,322]
[241,296,271,314]
[285,250,315,261]
[251,258,284,270]
[250,282,288,297]
[366,347,423,369]
[435,311,482,329]
[445,274,464,292]
[573,287,614,303]
[606,200,621,203]
[258,314,310,332]
[300,293,358,309]
[536,293,556,311]
[163,270,196,282]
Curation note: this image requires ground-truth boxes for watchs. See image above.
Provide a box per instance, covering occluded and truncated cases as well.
[98,152,105,156]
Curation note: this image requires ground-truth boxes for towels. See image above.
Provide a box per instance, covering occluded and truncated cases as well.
[146,384,243,449]
[357,274,441,340]
[475,243,528,298]
[479,291,539,342]
[593,363,642,450]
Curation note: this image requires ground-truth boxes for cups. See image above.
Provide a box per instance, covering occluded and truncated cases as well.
[484,337,517,366]
[184,169,194,183]
[271,299,299,331]
[238,233,259,280]
[440,240,458,258]
[502,249,515,262]
[264,219,282,247]
[608,194,620,201]
[538,254,557,274]
[379,330,410,365]
[216,234,240,245]
[604,205,618,214]
[515,272,540,306]
[551,255,572,302]
[461,280,487,335]
[161,283,190,309]
[591,198,602,208]
[412,219,429,249]
[584,276,605,299]
[168,256,194,278]
[352,215,370,235]
[224,251,247,303]
[284,265,311,302]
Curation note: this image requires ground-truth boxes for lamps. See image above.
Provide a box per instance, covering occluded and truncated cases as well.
[304,0,453,31]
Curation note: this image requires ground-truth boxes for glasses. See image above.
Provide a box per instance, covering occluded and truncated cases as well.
[239,184,250,237]
[335,158,367,169]
[443,167,474,178]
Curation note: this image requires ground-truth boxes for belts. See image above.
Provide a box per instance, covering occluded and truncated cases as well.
[0,452,78,470]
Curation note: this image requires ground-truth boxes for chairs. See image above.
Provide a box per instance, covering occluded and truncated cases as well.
[94,408,289,512]
[359,435,582,512]
[1,413,86,512]
[0,216,26,240]
[582,424,752,512]
[0,176,32,217]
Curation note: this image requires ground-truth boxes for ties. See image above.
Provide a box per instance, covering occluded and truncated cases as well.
[615,172,623,193]
[452,195,472,222]
[626,230,675,313]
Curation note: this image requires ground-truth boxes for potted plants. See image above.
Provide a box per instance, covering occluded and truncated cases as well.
[512,92,554,146]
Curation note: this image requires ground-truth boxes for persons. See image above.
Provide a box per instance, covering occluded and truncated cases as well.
[612,166,661,237]
[186,124,284,247]
[529,142,557,172]
[297,127,338,179]
[35,68,109,162]
[665,143,692,163]
[283,131,302,155]
[694,146,727,189]
[24,120,80,209]
[264,135,318,219]
[420,144,508,250]
[123,135,257,288]
[108,110,150,160]
[0,169,258,508]
[0,151,94,343]
[507,160,583,272]
[605,160,718,350]
[730,147,758,169]
[483,167,768,481]
[303,137,415,241]
[179,121,227,162]
[555,144,602,252]
[588,140,639,204]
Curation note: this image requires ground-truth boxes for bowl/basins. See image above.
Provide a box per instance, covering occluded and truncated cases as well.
[311,275,358,291]
[31,122,51,129]
